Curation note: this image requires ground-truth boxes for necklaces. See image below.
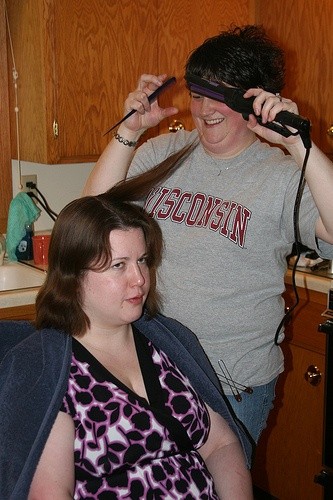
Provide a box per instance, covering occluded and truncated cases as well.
[203,139,257,176]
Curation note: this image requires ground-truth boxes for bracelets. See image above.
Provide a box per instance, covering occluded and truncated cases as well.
[113,133,140,148]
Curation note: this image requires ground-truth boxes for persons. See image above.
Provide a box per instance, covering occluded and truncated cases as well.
[83,25,333,476]
[0,128,254,500]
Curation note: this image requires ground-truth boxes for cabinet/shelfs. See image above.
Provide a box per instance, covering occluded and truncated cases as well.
[246,281,331,500]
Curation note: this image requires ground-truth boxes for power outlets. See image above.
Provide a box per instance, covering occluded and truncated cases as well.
[21,174,39,205]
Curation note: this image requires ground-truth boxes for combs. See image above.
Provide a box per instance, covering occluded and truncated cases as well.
[101,77,175,142]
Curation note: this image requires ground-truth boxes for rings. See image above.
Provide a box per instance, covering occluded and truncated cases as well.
[274,92,283,102]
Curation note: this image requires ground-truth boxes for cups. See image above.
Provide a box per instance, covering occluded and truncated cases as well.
[32,235,51,265]
